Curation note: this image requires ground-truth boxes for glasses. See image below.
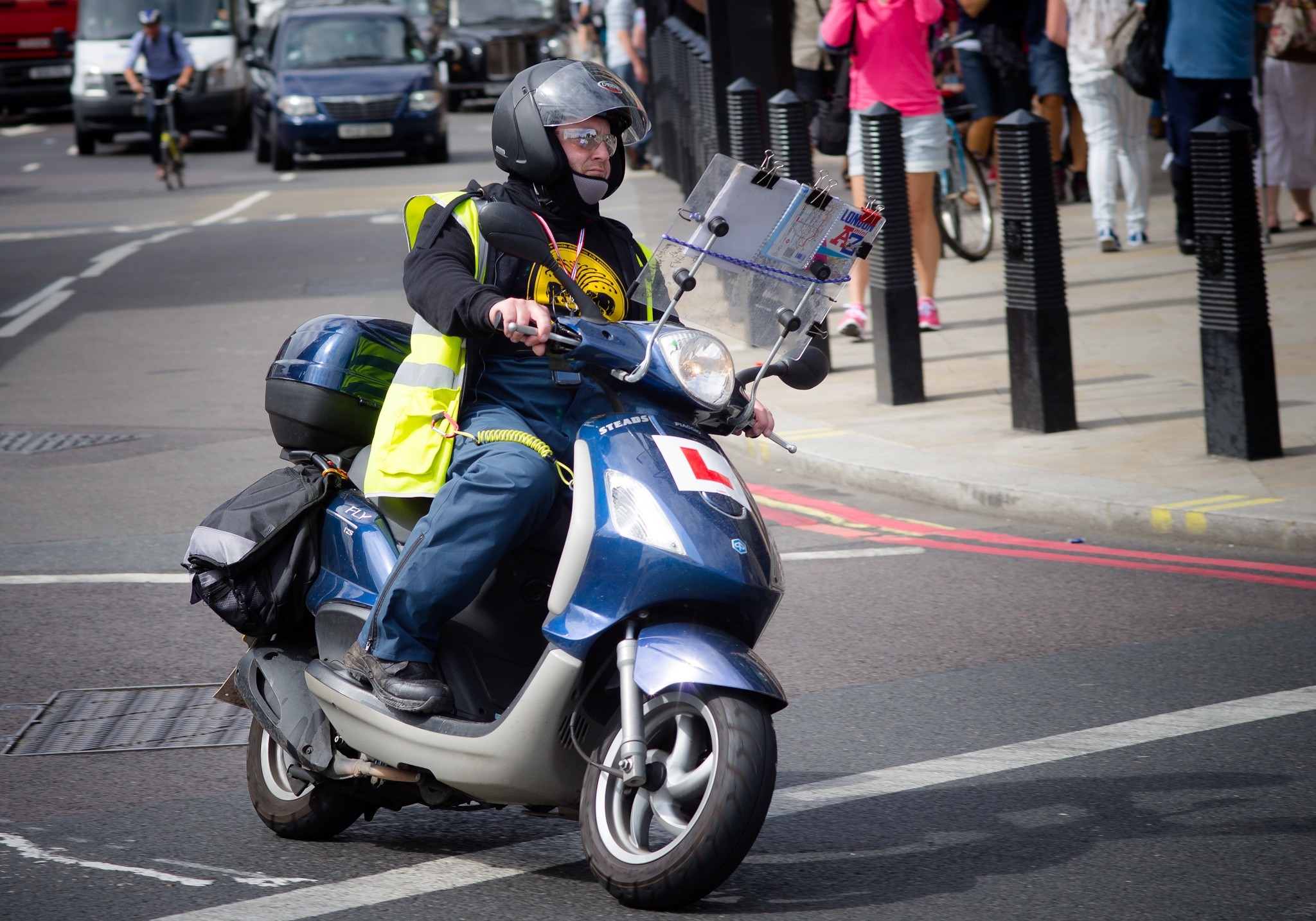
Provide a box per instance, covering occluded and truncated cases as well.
[554,127,618,156]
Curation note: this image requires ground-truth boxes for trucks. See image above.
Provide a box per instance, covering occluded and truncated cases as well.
[0,0,81,126]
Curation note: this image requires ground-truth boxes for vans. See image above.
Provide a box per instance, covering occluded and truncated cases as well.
[51,0,266,154]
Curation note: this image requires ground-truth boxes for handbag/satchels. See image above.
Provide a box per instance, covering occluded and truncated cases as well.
[182,458,331,638]
[1111,5,1148,76]
[1125,18,1159,104]
[818,58,852,155]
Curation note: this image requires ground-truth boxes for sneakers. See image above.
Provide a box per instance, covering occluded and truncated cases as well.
[343,638,456,718]
[1099,229,1121,252]
[1125,232,1148,246]
[840,304,867,341]
[916,298,941,330]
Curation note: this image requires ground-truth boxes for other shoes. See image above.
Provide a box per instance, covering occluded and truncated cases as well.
[178,133,190,149]
[1051,173,1067,202]
[1072,178,1089,203]
[1268,220,1280,233]
[1149,116,1167,140]
[155,163,166,178]
[957,155,997,208]
[1178,233,1197,254]
[1299,218,1313,227]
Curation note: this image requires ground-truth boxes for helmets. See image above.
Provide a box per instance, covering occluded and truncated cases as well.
[491,56,651,211]
[138,8,160,25]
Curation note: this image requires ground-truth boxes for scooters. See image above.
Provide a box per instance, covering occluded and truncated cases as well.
[214,197,833,907]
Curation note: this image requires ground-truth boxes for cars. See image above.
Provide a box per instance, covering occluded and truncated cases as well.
[249,1,439,53]
[241,7,457,172]
[439,0,579,113]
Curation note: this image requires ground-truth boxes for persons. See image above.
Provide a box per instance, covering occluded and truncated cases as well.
[1028,18,1090,202]
[1144,0,1258,255]
[342,57,775,712]
[955,0,1046,207]
[124,9,194,180]
[815,0,950,338]
[568,0,604,61]
[1044,0,1154,251]
[1251,0,1316,233]
[603,0,652,170]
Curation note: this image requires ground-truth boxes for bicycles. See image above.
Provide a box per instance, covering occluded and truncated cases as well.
[932,30,995,261]
